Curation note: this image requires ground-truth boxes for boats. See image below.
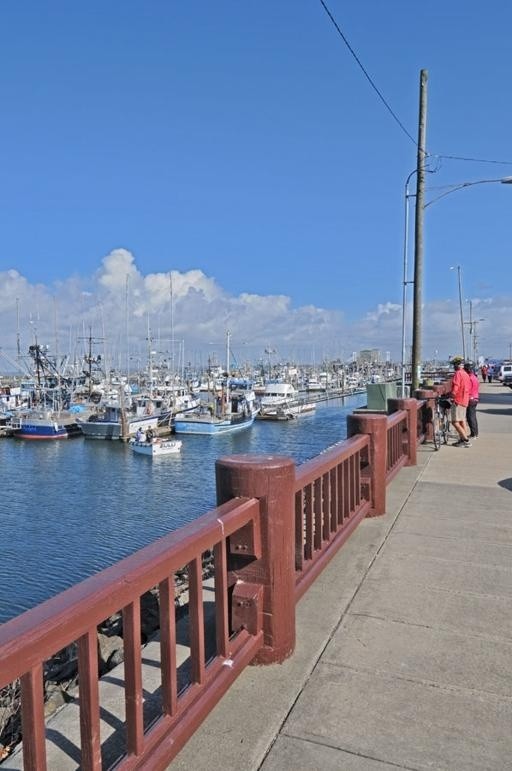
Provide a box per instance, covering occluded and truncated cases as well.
[127,437,183,456]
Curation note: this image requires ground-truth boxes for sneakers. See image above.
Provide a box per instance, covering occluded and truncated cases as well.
[451,434,479,448]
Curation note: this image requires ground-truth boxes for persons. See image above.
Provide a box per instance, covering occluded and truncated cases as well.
[136,426,153,443]
[464,364,479,440]
[481,365,493,383]
[441,356,471,447]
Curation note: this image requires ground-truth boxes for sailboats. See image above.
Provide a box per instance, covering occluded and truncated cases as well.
[0,273,451,440]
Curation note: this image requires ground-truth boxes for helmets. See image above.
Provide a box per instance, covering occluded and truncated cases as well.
[463,361,476,369]
[449,356,464,365]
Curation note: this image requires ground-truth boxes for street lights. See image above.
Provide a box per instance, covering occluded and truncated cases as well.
[411,175,512,401]
